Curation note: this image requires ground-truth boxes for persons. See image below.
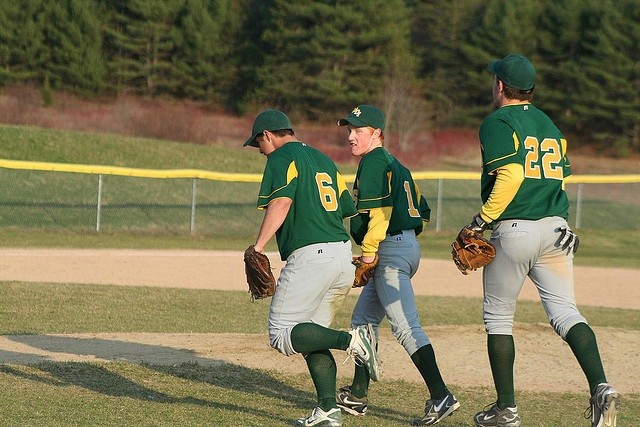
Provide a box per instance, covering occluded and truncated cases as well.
[467,53,621,427]
[244,110,384,427]
[336,104,461,427]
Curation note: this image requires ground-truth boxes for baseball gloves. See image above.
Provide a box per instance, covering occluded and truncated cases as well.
[243,244,276,303]
[450,226,496,275]
[352,254,379,288]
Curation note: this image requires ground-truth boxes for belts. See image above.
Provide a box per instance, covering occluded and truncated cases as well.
[391,230,403,237]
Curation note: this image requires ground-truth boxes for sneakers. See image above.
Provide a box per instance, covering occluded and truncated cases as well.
[336,385,368,417]
[297,406,344,427]
[583,383,621,427]
[342,324,380,383]
[473,403,521,427]
[417,394,461,426]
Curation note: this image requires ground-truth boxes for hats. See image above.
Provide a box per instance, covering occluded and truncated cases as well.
[337,104,385,133]
[487,53,537,90]
[243,109,293,147]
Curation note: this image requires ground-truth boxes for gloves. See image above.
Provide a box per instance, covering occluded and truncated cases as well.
[554,224,580,256]
